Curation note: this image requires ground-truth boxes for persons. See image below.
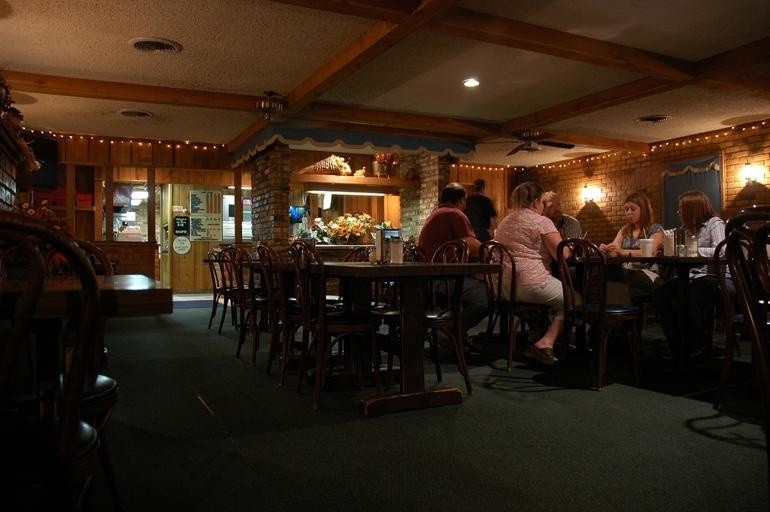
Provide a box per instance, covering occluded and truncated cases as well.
[463,179,499,243]
[541,190,582,241]
[418,182,496,356]
[654,190,737,372]
[598,193,664,351]
[487,182,583,367]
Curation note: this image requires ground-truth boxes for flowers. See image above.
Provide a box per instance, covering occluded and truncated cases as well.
[312,210,376,238]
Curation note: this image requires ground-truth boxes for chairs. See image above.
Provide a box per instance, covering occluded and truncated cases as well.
[252,245,296,375]
[311,245,369,357]
[725,203,769,420]
[208,250,238,335]
[479,240,555,372]
[220,244,267,337]
[555,237,641,393]
[3,236,119,415]
[0,208,126,511]
[283,239,385,411]
[375,240,473,399]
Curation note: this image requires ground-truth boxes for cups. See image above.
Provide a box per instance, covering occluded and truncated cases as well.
[639,238,656,257]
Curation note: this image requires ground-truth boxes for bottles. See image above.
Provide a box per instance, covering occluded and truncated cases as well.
[664,229,699,257]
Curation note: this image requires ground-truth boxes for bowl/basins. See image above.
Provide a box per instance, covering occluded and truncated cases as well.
[698,247,725,258]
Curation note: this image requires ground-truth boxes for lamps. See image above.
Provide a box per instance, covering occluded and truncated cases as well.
[515,125,543,153]
[256,88,287,122]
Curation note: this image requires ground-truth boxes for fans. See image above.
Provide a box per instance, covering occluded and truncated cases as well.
[505,132,575,156]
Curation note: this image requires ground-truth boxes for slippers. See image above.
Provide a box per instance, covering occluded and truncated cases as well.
[521,342,560,370]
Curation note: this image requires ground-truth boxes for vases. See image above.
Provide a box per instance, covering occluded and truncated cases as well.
[336,237,370,245]
